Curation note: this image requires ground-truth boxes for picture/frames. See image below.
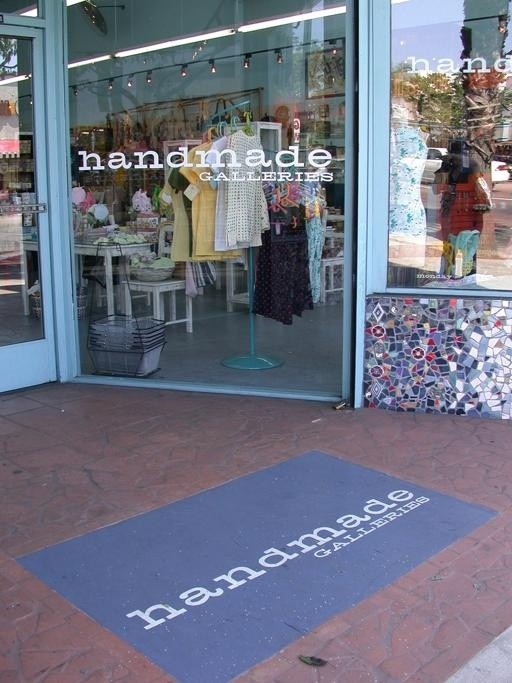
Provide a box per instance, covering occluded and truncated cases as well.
[303,48,344,99]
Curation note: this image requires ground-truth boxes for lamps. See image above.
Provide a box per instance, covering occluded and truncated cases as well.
[67,9,299,67]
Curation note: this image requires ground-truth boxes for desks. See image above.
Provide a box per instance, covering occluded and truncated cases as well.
[19,231,154,317]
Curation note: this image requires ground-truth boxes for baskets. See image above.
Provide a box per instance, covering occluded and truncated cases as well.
[130,264,176,283]
[87,311,170,379]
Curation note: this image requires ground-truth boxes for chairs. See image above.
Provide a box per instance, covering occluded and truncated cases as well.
[121,220,195,333]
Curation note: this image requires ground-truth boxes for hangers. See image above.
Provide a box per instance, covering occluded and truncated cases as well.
[263,164,326,226]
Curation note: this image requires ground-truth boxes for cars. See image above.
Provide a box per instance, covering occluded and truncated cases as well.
[400,148,511,191]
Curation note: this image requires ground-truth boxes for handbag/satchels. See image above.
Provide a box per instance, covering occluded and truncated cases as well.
[473,176,493,212]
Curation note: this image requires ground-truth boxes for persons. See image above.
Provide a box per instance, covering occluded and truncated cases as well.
[430,139,494,277]
